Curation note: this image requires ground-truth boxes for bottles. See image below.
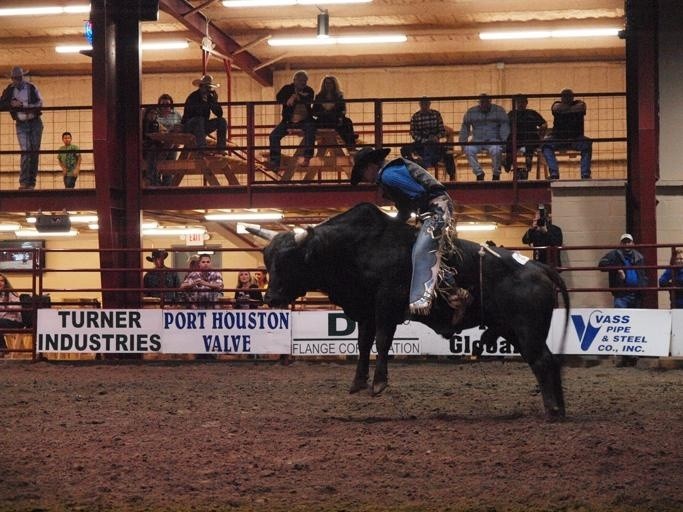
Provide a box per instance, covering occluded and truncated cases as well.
[191,75,220,89]
[145,249,168,263]
[186,254,199,264]
[619,232,633,243]
[4,65,30,79]
[350,145,391,185]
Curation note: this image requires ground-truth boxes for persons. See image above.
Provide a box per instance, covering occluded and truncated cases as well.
[181,74,227,157]
[504,94,547,173]
[55,132,81,189]
[298,76,356,168]
[141,105,168,186]
[350,146,474,328]
[18,293,50,329]
[521,204,564,271]
[598,233,649,370]
[232,270,263,360]
[263,70,314,173]
[179,254,223,360]
[183,255,200,281]
[0,272,23,362]
[155,94,182,186]
[458,93,511,182]
[253,266,270,309]
[541,89,593,180]
[659,248,682,308]
[142,248,180,303]
[0,66,42,189]
[408,96,456,183]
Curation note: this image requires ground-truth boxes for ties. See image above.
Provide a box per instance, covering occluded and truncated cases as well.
[0,239,45,273]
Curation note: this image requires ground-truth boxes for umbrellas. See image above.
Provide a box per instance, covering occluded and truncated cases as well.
[446,287,474,326]
[18,183,35,190]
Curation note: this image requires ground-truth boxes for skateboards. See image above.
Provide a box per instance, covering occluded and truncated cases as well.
[246,202,570,423]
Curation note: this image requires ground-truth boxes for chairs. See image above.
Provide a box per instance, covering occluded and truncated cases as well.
[476,173,500,181]
[447,172,601,179]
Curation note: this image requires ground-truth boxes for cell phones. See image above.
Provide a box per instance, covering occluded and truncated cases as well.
[537,207,545,227]
